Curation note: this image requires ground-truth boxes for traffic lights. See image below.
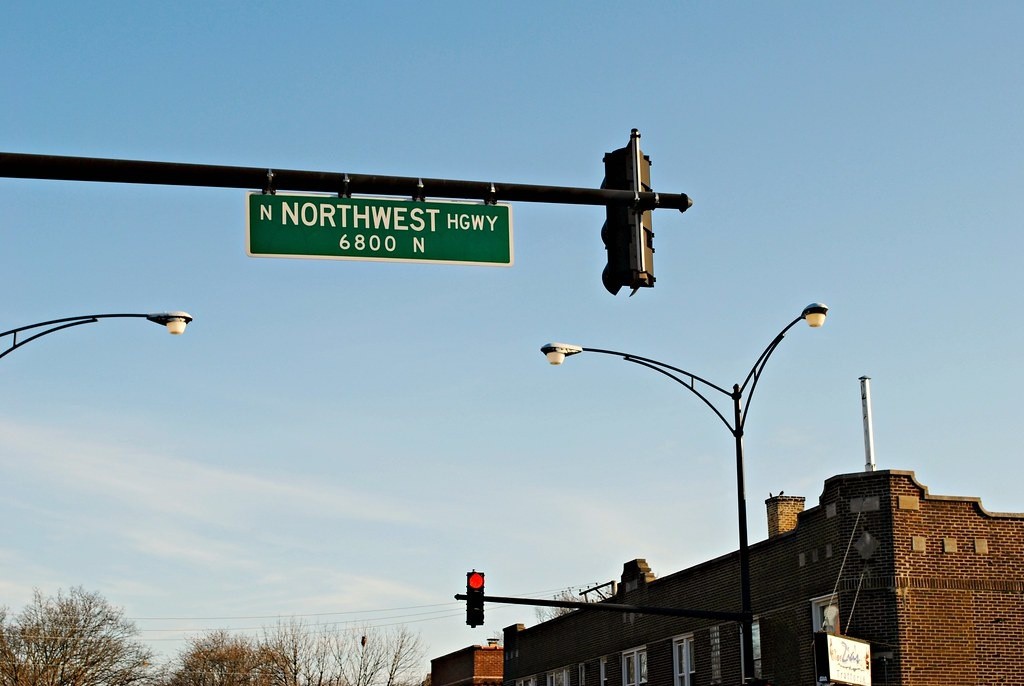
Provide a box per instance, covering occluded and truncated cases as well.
[467,571,485,628]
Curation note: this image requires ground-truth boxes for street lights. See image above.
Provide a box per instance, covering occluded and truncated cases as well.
[0,311,194,360]
[540,302,828,686]
